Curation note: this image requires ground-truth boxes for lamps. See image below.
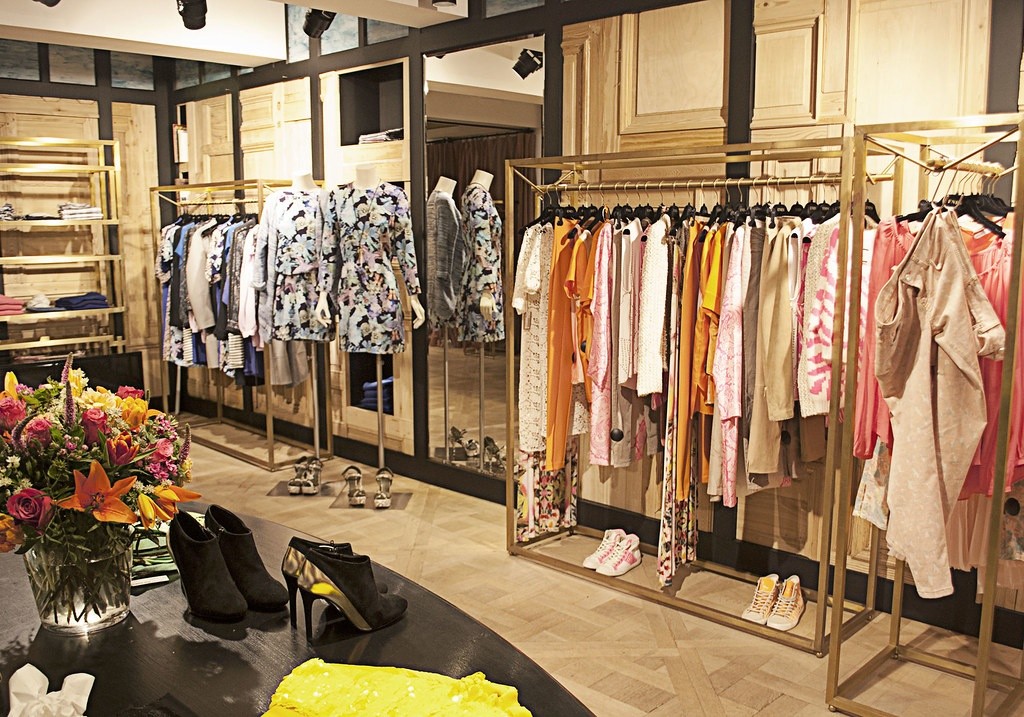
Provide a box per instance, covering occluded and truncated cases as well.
[175,0,209,31]
[302,7,337,40]
[511,48,543,79]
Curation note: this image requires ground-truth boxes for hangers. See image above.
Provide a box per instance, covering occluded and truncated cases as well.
[517,158,1015,246]
[170,200,260,237]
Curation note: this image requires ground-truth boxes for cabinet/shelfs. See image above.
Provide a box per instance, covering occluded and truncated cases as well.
[1,137,126,353]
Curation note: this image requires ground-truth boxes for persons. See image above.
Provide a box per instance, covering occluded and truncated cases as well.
[427,170,506,351]
[255,163,426,355]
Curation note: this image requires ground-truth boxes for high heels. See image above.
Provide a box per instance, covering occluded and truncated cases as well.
[169,508,248,623]
[287,456,309,494]
[281,536,388,624]
[301,457,323,494]
[448,426,480,456]
[374,467,393,507]
[342,465,366,504]
[483,436,526,479]
[204,504,289,608]
[297,547,408,642]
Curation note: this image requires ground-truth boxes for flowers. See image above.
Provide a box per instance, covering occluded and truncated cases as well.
[0,348,204,624]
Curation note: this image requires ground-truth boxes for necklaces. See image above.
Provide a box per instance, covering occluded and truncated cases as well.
[350,178,381,267]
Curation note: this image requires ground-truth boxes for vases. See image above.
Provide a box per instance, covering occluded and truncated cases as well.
[18,516,132,636]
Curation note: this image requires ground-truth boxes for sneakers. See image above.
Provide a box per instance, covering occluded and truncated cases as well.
[767,575,805,631]
[583,529,626,569]
[742,574,781,625]
[596,534,642,576]
